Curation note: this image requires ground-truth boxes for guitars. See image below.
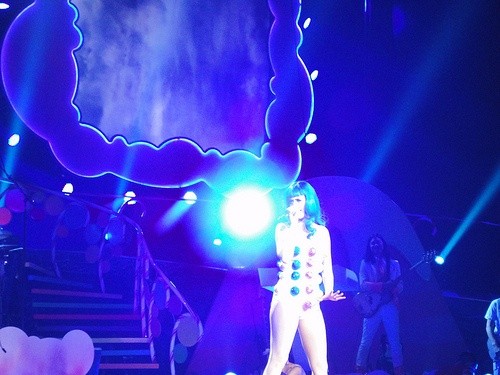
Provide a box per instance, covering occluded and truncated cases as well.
[352,252,438,317]
[488,337,500,361]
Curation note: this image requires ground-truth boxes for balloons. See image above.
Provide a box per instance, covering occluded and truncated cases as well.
[43,197,63,216]
[173,343,188,364]
[177,315,199,347]
[7,190,25,213]
[83,222,101,265]
[0,207,12,225]
[0,326,94,374]
[167,297,183,315]
[151,319,161,337]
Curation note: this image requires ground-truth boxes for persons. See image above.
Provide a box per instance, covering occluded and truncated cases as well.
[355,231,404,375]
[263,348,306,375]
[262,179,346,375]
[484,297,500,375]
